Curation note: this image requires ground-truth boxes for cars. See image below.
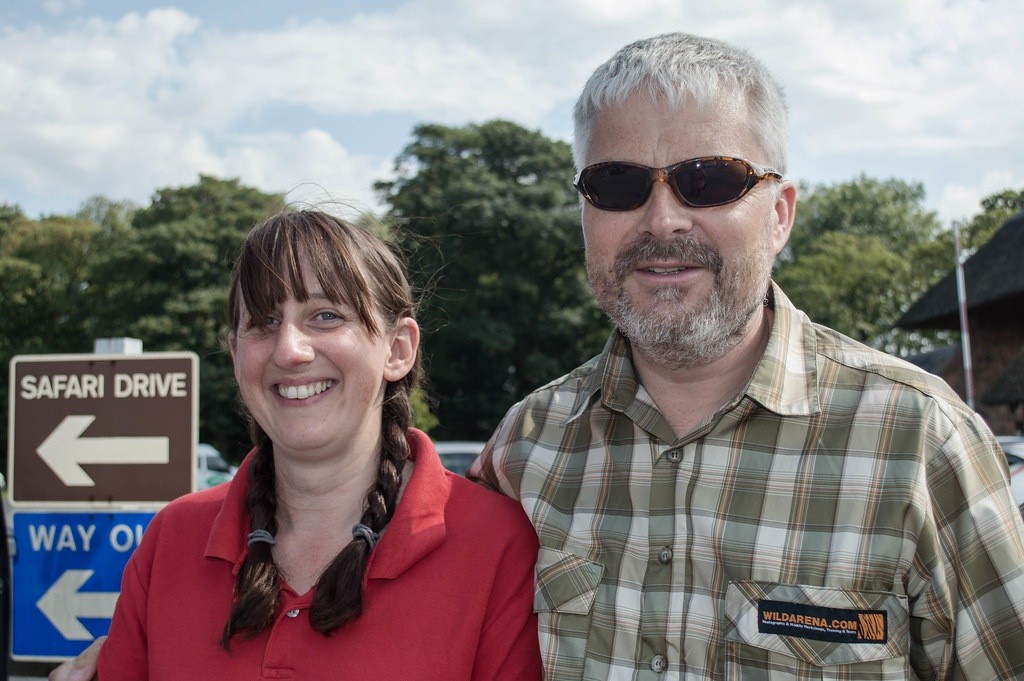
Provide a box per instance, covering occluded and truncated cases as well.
[992,434,1024,517]
[434,440,489,479]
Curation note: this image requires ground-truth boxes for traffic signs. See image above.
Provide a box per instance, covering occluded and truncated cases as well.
[6,354,196,506]
[9,509,163,658]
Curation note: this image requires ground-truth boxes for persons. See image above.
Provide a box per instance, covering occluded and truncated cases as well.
[49,32,1024,681]
[97,181,542,681]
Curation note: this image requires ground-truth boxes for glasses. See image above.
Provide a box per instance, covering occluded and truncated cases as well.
[572,155,785,212]
